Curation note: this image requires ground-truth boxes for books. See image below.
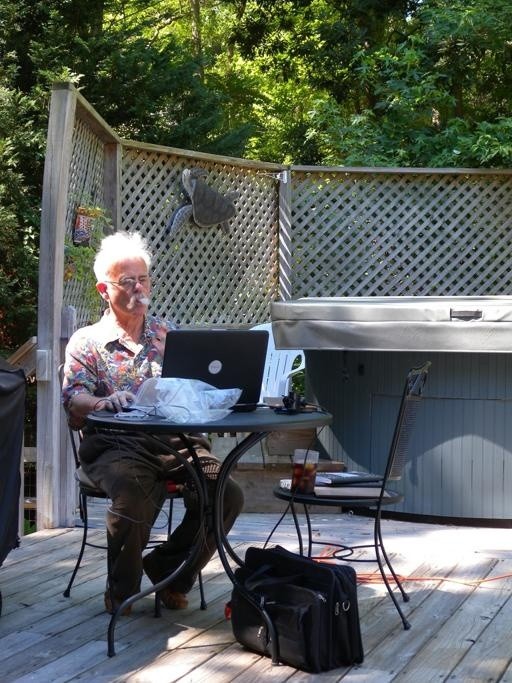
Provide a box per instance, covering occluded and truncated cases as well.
[313,480,391,499]
[315,470,384,484]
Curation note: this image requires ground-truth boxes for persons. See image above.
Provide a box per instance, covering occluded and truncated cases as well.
[61,228,245,617]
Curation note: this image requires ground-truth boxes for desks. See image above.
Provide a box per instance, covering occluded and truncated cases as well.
[85,405,332,657]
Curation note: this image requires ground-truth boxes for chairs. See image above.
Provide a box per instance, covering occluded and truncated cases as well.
[56,364,207,616]
[262,362,431,631]
[249,323,305,408]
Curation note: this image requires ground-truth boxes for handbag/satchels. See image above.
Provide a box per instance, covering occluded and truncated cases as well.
[227,544,365,674]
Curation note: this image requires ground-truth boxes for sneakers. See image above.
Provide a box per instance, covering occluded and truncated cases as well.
[104,577,133,617]
[142,546,189,610]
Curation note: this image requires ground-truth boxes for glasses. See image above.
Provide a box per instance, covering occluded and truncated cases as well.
[101,276,152,292]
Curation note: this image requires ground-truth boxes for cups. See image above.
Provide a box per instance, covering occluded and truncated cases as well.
[290,447,320,497]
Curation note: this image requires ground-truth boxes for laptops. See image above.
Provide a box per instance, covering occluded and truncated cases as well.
[161,329,269,412]
